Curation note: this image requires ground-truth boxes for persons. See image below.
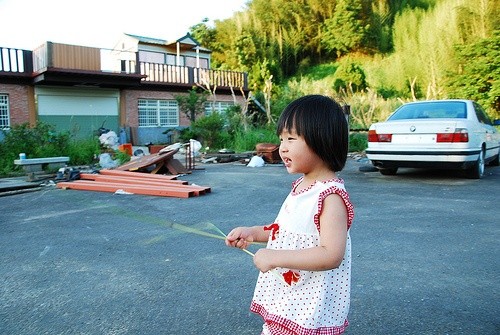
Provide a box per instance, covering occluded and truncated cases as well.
[226,94,354,334]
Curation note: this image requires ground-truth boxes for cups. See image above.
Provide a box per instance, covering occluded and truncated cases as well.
[20,154,25,163]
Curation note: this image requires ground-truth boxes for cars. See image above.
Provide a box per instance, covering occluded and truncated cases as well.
[365,99,500,179]
